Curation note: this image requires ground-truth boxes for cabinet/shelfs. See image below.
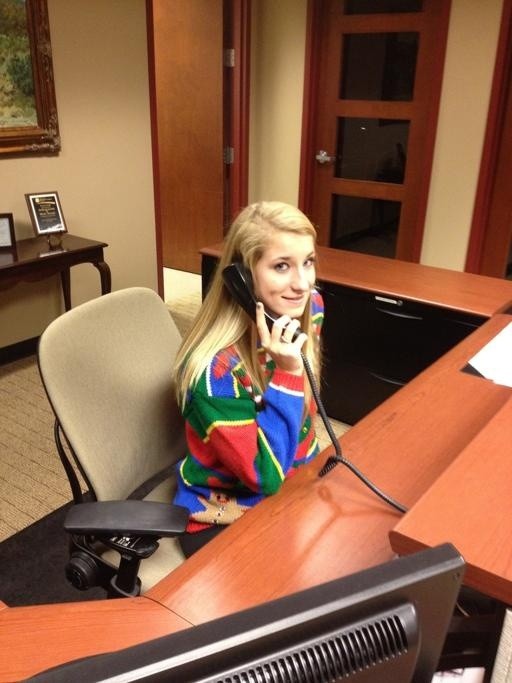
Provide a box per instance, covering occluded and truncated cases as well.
[200,236,512,424]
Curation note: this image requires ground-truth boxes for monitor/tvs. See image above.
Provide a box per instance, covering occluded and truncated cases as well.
[20,542,466,683]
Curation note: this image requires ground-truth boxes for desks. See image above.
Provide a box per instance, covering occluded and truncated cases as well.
[138,312,512,626]
[0,232,112,312]
[0,596,192,683]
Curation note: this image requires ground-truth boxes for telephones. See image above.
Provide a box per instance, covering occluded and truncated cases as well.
[221,263,303,343]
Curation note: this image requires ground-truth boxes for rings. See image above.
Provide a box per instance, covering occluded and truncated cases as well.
[280,336,289,345]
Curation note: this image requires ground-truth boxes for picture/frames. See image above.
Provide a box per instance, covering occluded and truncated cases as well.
[0,212,17,250]
[25,189,69,235]
[2,0,62,157]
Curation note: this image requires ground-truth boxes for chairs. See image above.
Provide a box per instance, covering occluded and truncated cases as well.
[39,286,194,595]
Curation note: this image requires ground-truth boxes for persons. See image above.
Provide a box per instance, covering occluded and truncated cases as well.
[169,200,325,560]
